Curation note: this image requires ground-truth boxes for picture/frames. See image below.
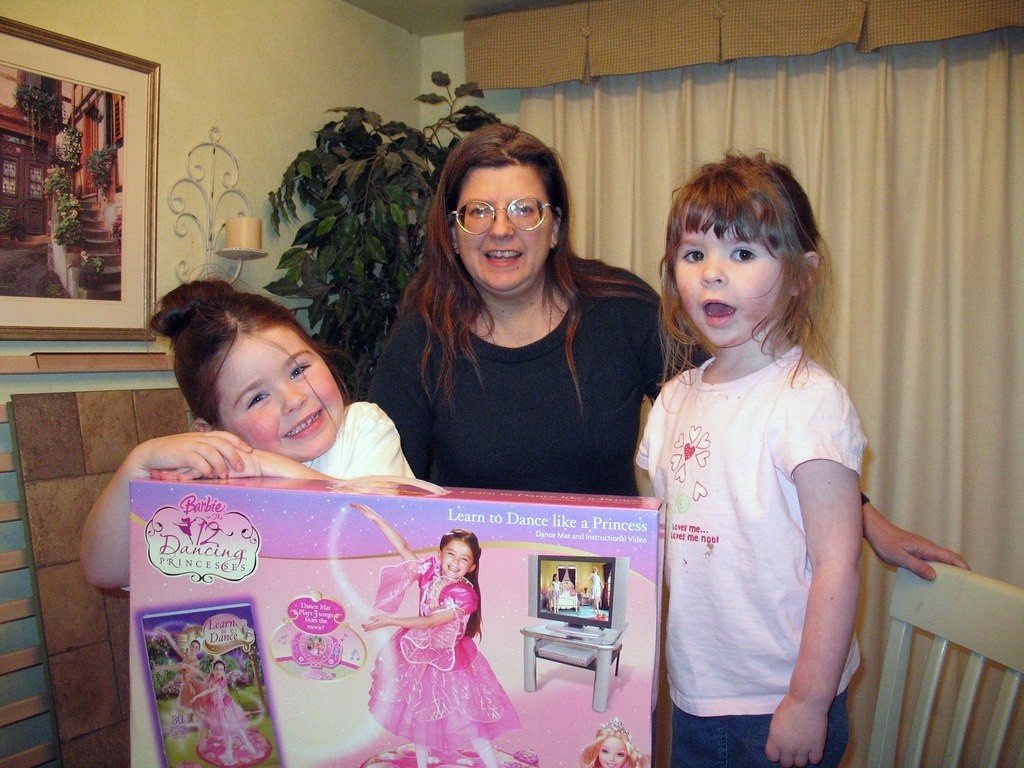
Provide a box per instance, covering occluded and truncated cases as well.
[0,16,163,342]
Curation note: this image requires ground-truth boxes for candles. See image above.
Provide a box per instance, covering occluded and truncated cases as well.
[225,212,262,249]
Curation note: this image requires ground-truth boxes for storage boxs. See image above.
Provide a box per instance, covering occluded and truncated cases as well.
[128,465,667,766]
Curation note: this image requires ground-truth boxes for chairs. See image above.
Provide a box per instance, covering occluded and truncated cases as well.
[865,560,1023,768]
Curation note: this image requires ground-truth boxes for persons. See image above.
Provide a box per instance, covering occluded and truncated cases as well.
[580,566,604,613]
[634,152,867,768]
[542,574,569,614]
[365,124,971,580]
[152,627,256,761]
[349,503,523,768]
[79,279,415,597]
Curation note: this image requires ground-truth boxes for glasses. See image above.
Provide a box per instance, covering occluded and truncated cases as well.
[447,196,554,236]
